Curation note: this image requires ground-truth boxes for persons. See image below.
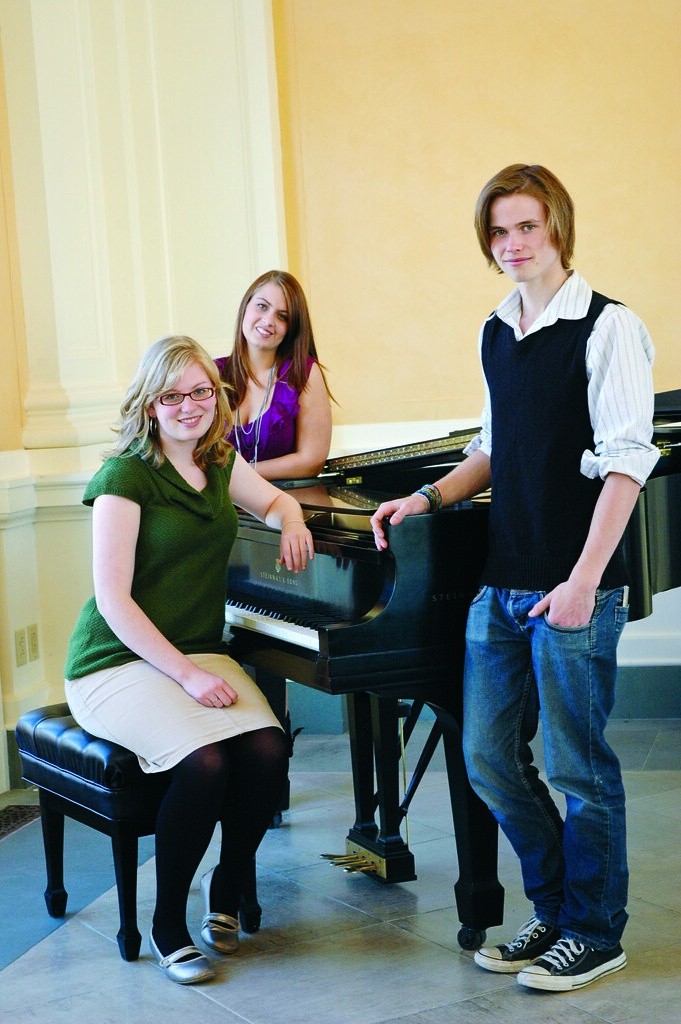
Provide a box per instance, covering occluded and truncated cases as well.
[65,333,314,986]
[208,270,343,481]
[370,162,659,990]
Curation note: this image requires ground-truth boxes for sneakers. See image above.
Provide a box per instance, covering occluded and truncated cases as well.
[517,935,626,991]
[474,915,563,973]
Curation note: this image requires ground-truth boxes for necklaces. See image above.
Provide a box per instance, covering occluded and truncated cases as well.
[234,358,278,469]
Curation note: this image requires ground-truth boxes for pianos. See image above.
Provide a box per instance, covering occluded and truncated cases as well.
[215,390,679,950]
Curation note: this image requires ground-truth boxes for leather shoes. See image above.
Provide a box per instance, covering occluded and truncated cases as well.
[200,864,240,953]
[148,925,216,984]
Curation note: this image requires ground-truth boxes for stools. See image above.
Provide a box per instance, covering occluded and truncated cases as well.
[16,704,262,963]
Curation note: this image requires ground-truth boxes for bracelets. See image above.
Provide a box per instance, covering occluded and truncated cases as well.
[282,520,305,527]
[412,484,443,514]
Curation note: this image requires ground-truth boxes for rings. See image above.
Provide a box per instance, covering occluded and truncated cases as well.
[212,698,219,703]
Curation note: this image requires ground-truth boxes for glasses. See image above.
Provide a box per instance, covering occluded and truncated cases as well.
[156,385,217,406]
